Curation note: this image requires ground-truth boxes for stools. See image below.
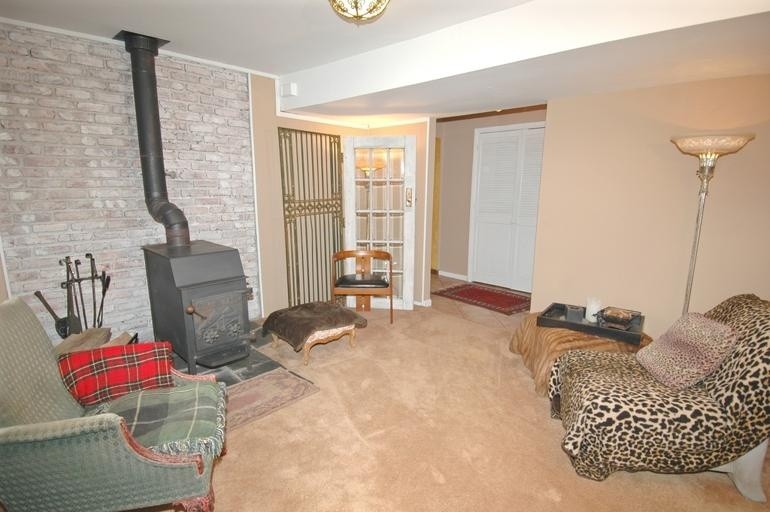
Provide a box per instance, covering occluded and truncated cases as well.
[262,301,369,366]
[524,313,653,398]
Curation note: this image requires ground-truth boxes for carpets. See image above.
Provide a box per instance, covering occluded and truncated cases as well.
[224,367,321,435]
[430,281,531,317]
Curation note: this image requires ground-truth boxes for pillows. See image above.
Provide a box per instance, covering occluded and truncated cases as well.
[54,340,173,408]
[636,310,738,392]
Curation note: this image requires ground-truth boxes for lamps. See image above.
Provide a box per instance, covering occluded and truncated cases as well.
[328,0,390,26]
[669,133,756,316]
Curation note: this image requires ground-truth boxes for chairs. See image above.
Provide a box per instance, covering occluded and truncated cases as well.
[0,296,229,512]
[548,291,770,482]
[330,250,394,324]
